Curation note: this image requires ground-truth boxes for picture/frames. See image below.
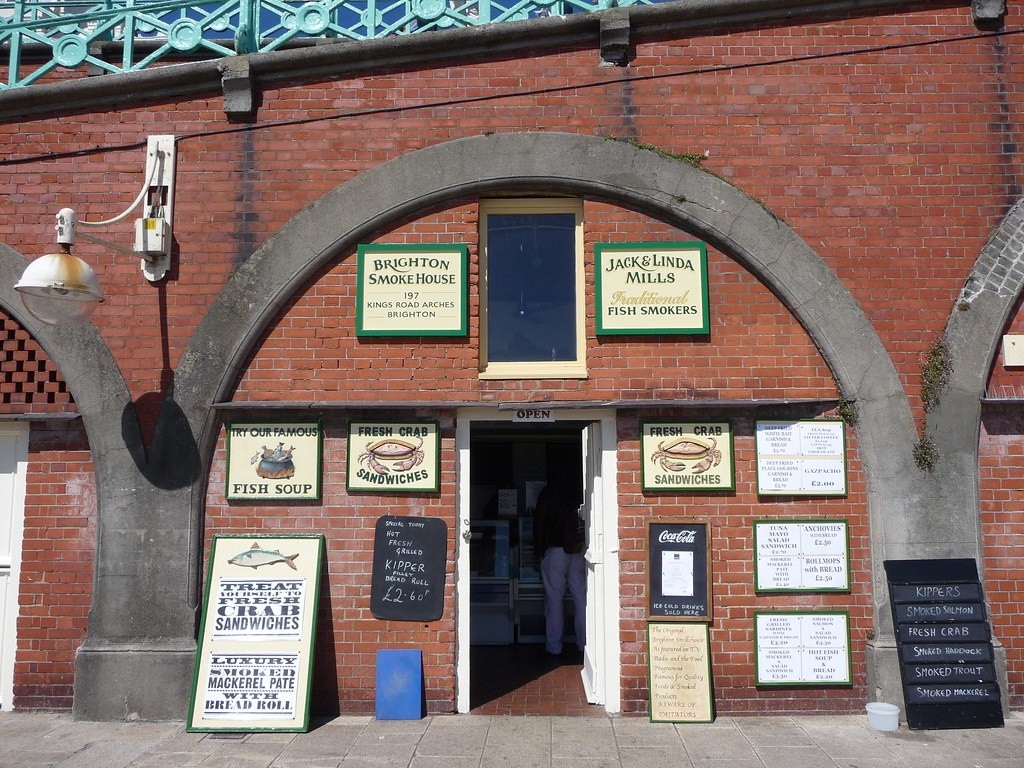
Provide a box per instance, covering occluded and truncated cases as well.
[645,518,713,622]
[754,610,853,688]
[755,417,848,497]
[753,518,851,594]
[186,533,323,733]
[356,243,469,337]
[593,240,710,335]
[345,419,442,492]
[647,622,714,723]
[639,418,736,492]
[224,417,322,501]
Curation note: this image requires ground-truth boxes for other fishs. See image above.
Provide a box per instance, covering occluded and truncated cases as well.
[250,442,296,466]
[228,541,300,572]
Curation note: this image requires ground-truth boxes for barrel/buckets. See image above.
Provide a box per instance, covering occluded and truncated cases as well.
[867,702,900,731]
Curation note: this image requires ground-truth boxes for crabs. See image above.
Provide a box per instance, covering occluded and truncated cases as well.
[360,437,423,474]
[651,437,722,474]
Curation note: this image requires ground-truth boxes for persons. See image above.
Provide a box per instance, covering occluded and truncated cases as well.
[533,463,586,663]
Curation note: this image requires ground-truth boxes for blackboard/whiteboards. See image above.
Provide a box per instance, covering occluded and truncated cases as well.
[647,622,713,724]
[645,519,712,621]
[883,558,1006,730]
[369,515,448,623]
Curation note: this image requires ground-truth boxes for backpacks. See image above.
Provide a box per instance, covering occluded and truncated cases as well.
[560,504,585,554]
